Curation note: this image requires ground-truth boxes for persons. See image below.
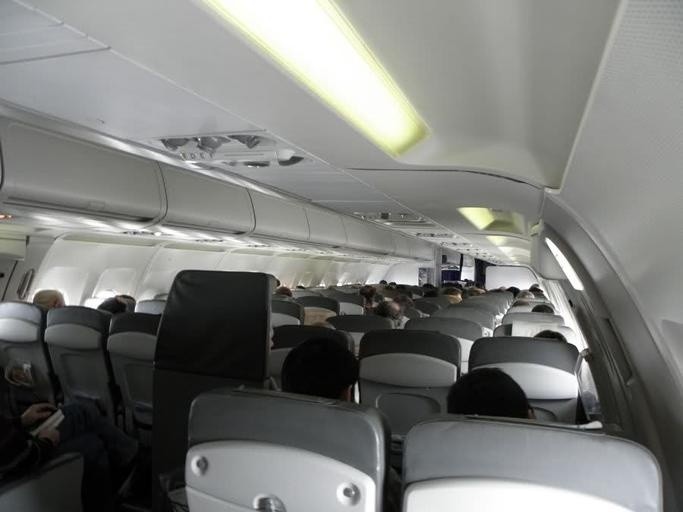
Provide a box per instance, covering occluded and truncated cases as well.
[447,368,534,418]
[0,396,139,469]
[422,278,553,312]
[376,301,401,320]
[275,288,291,297]
[97,297,126,313]
[360,279,396,306]
[281,335,358,402]
[535,330,566,342]
[33,290,64,309]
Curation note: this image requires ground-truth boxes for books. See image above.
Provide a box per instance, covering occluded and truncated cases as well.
[29,409,64,438]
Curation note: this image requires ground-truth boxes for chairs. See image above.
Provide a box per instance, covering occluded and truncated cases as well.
[0,284,662,512]
